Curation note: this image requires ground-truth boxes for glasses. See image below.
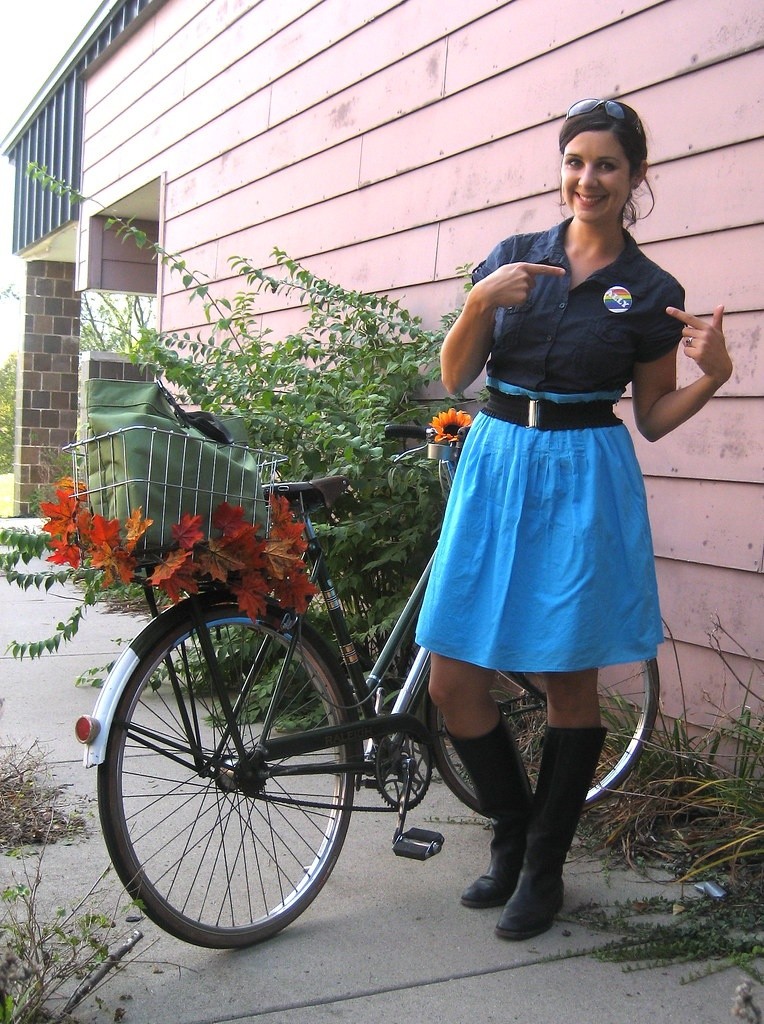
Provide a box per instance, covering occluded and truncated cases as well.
[564,98,645,136]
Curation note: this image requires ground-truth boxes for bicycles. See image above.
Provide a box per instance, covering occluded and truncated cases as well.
[74,422,659,950]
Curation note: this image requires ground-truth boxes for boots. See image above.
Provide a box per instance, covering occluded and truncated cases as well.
[444,700,534,909]
[497,724,608,941]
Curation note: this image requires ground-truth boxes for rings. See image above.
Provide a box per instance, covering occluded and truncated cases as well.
[686,337,693,346]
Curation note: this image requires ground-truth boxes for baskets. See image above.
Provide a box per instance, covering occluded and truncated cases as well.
[61,425,290,564]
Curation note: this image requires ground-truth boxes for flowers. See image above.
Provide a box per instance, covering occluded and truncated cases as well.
[429,408,472,445]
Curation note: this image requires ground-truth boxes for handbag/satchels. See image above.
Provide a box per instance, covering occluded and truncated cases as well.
[86,378,272,548]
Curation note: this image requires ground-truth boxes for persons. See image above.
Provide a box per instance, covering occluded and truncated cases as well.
[413,99,732,939]
